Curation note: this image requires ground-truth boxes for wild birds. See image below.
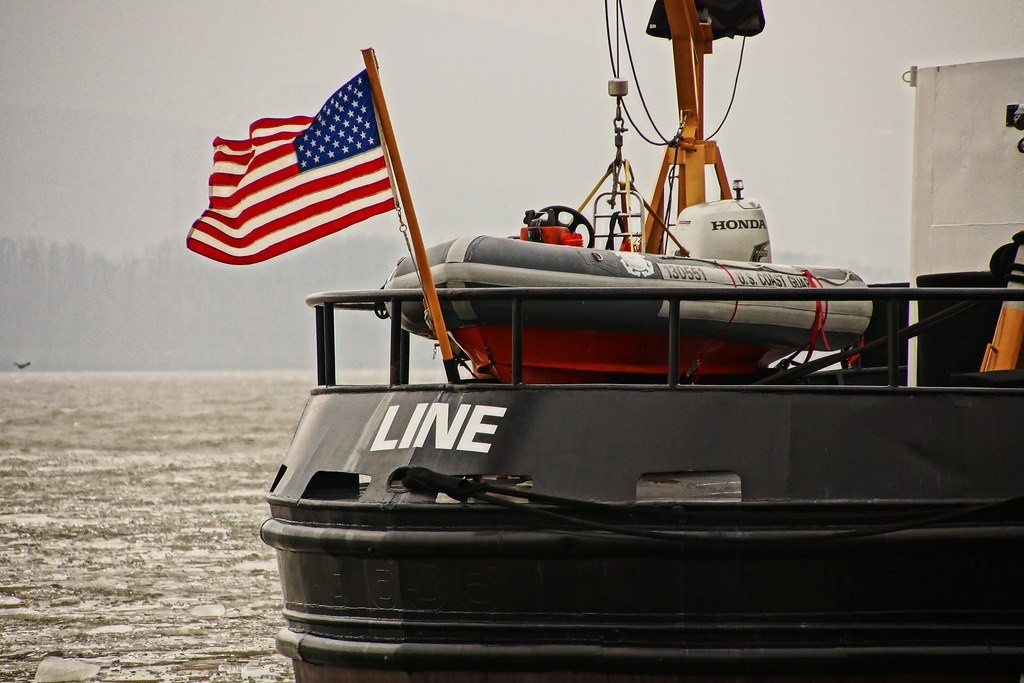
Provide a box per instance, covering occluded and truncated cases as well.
[13,361,31,369]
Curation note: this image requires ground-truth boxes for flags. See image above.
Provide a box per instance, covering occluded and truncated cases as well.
[185,70,398,266]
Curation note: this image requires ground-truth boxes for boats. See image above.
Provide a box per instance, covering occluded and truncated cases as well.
[256,0,1024,683]
